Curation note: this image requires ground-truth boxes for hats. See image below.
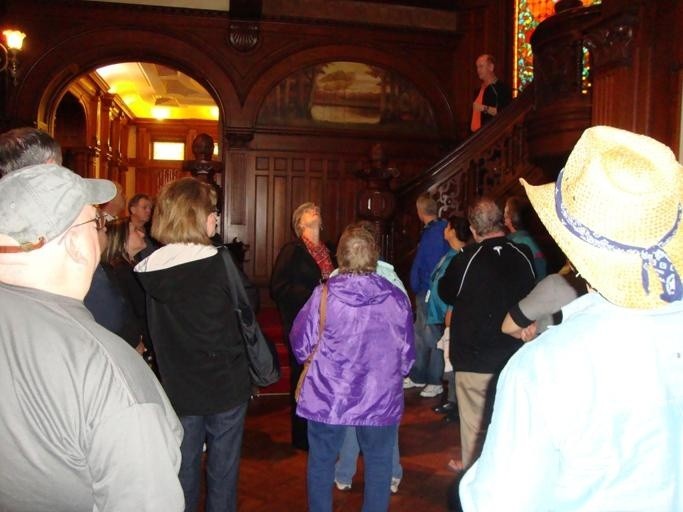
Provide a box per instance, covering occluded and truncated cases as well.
[0,164,117,252]
[520,126,683,309]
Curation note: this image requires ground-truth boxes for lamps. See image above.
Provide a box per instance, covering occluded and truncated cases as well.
[0,24,29,88]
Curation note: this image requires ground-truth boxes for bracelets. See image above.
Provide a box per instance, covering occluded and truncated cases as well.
[482,106,489,114]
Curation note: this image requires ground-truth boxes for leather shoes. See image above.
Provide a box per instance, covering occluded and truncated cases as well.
[431,401,459,424]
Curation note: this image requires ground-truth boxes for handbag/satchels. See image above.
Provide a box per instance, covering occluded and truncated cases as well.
[238,305,280,387]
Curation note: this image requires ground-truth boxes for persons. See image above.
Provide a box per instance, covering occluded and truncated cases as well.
[132,176,254,512]
[290,230,411,511]
[0,126,62,177]
[90,194,170,378]
[411,196,546,423]
[457,122,680,512]
[328,223,411,493]
[469,54,510,132]
[501,260,587,341]
[269,202,341,451]
[0,163,188,510]
[437,195,537,473]
[178,132,223,178]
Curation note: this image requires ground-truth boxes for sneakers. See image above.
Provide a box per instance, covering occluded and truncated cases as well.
[403,375,444,399]
[334,478,352,490]
[390,477,401,493]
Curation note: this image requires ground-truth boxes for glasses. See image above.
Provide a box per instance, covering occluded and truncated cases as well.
[74,207,106,229]
